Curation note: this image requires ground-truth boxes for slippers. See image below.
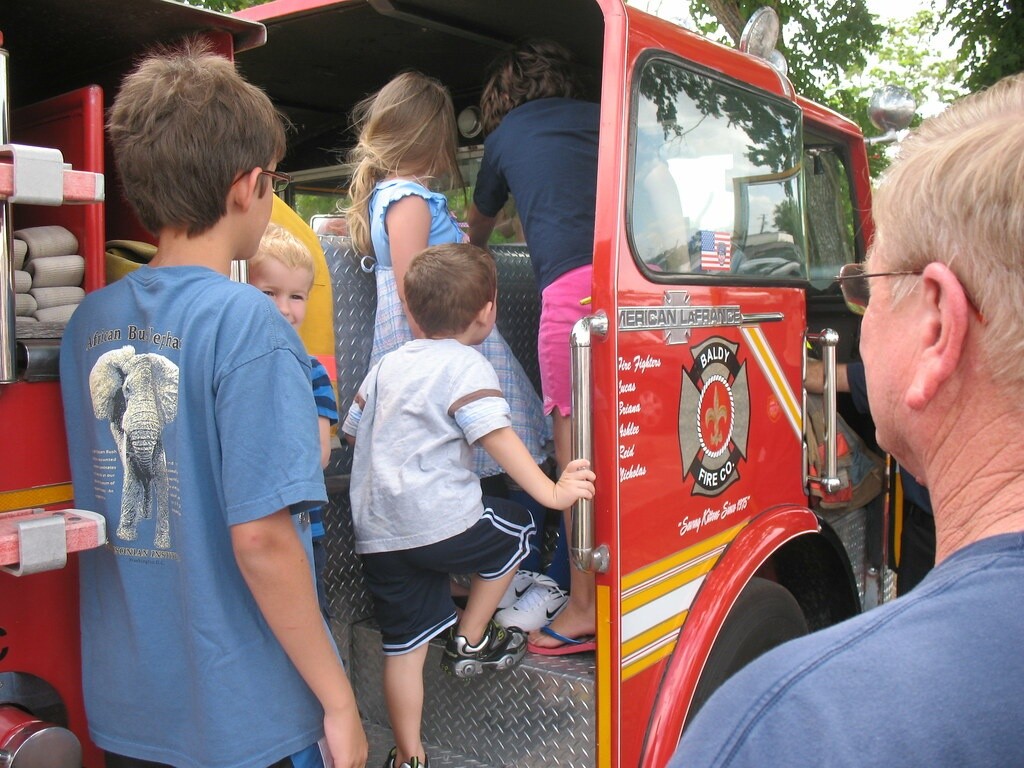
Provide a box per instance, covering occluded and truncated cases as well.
[527,623,597,655]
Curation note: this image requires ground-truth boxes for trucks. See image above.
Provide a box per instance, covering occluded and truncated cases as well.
[0,1,919,768]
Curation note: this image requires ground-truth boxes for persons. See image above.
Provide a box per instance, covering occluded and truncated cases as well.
[806,361,937,599]
[342,242,595,768]
[58,52,368,767]
[466,39,692,654]
[352,73,555,600]
[664,74,1024,768]
[250,221,339,625]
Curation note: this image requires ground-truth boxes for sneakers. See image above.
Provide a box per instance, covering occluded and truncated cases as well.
[439,618,527,679]
[496,570,539,609]
[495,573,571,632]
[383,746,431,768]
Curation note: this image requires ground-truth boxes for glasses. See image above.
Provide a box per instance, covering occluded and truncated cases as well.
[834,263,988,327]
[233,170,290,192]
